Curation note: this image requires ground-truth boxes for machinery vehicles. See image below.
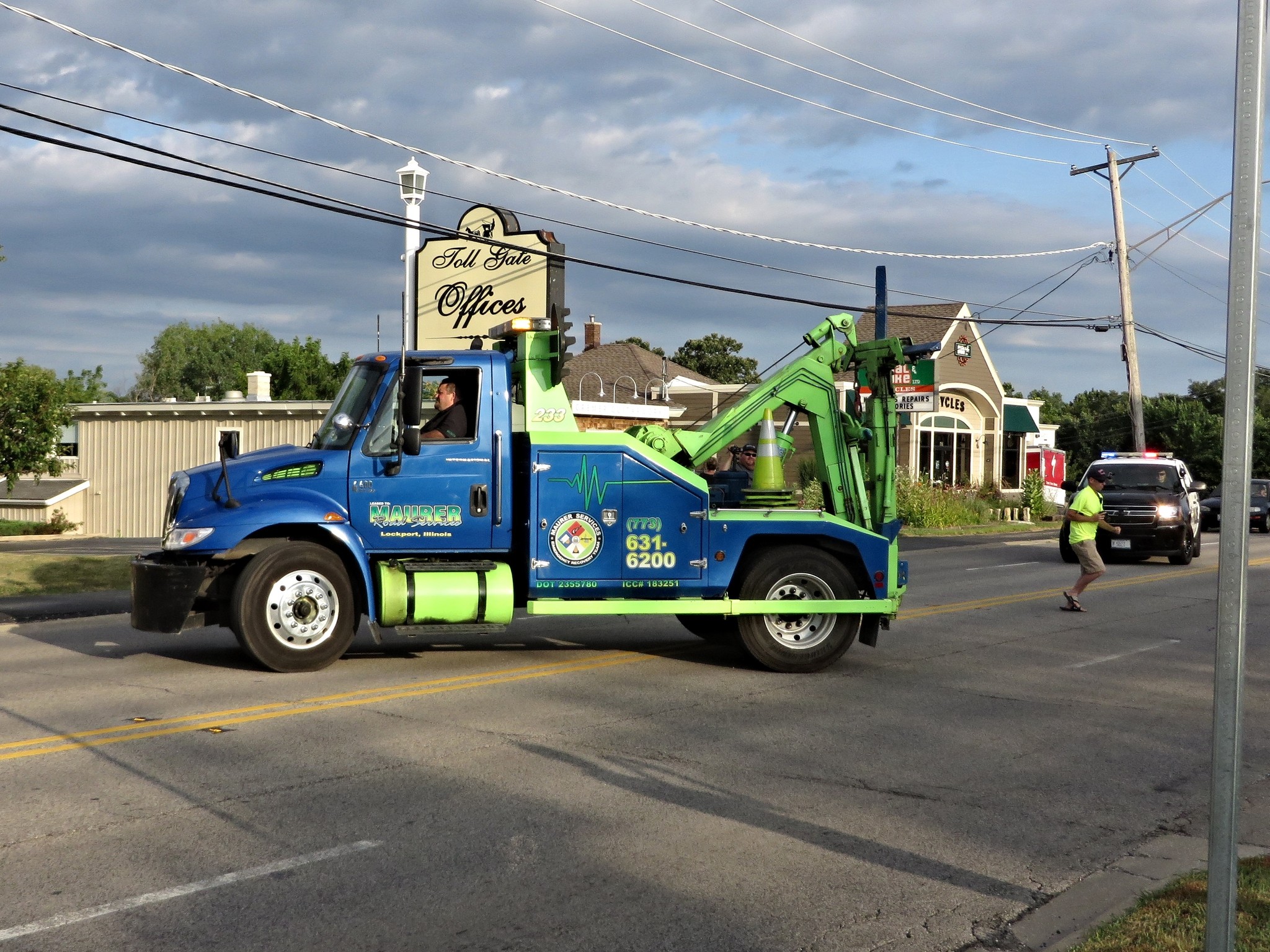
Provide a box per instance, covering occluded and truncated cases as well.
[123,309,942,678]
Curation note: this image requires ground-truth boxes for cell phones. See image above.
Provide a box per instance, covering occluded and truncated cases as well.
[1099,510,1106,515]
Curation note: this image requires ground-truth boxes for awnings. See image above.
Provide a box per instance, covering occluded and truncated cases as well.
[1003,404,1039,432]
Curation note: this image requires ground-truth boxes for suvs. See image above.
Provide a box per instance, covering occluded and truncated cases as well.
[1056,447,1207,567]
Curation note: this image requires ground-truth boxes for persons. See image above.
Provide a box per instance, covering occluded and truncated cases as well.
[1149,468,1173,488]
[723,444,756,485]
[1058,465,1121,612]
[699,457,719,483]
[420,378,472,439]
[1255,485,1267,496]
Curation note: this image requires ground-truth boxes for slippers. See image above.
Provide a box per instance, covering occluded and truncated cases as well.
[1059,606,1081,611]
[1063,590,1081,609]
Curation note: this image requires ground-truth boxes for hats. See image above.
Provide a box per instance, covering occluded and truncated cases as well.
[706,457,718,470]
[1258,484,1266,490]
[743,443,757,453]
[1087,466,1107,481]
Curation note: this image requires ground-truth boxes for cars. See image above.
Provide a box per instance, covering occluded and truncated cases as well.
[1198,477,1270,533]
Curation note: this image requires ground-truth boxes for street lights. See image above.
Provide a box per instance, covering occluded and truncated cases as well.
[396,153,431,351]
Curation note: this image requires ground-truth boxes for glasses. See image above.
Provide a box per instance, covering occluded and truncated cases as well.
[742,452,757,457]
[1155,473,1165,476]
[1096,480,1105,483]
